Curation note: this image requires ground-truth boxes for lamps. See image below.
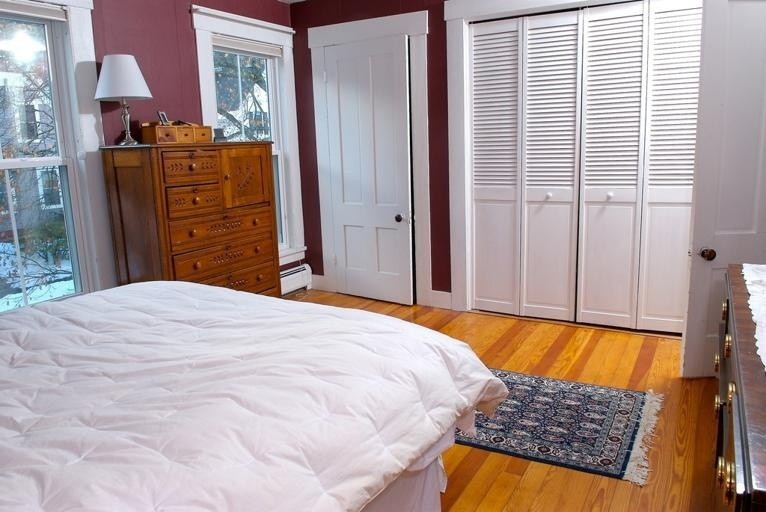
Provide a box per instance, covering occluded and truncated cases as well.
[94,54,152,145]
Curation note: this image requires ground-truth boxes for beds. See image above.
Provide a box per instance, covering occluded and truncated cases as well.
[0,280,508,512]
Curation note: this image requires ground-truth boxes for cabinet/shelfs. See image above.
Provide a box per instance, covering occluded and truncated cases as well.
[711,263,766,512]
[100,142,282,299]
[575,0,704,332]
[444,0,578,331]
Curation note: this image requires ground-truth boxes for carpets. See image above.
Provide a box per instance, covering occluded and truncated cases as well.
[455,368,665,487]
